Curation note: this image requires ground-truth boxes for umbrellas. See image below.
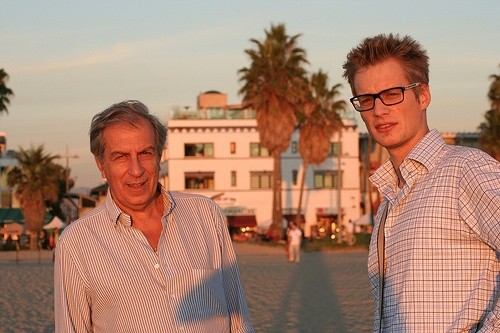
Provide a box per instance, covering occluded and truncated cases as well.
[43,215,68,258]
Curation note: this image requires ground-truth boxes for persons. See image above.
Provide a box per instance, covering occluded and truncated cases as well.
[343,33,500,333]
[265,218,356,263]
[54,101,253,333]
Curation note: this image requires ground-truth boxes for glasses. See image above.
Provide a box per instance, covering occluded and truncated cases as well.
[350,81,424,112]
